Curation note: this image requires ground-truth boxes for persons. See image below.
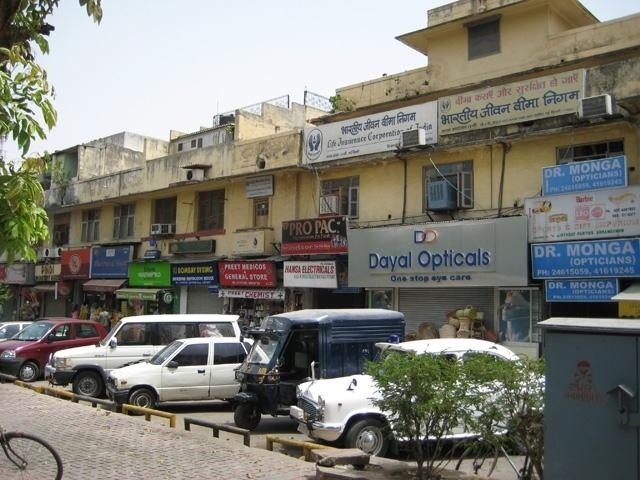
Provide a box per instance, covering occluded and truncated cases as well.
[71,301,113,332]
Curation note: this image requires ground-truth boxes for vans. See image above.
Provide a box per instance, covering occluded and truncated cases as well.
[0,314,109,382]
[45,313,244,399]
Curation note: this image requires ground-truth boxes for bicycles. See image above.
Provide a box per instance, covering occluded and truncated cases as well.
[0,425,63,480]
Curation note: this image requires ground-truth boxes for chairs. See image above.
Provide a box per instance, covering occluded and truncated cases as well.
[280,340,309,380]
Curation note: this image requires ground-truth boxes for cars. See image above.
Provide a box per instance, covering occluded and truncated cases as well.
[105,337,274,416]
[290,338,546,457]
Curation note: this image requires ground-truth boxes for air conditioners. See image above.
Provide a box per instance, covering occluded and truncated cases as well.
[43,248,68,258]
[186,169,204,183]
[579,93,612,119]
[427,180,458,209]
[151,224,175,234]
[402,128,427,148]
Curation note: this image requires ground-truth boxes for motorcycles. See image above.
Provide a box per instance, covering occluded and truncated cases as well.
[234,309,407,431]
